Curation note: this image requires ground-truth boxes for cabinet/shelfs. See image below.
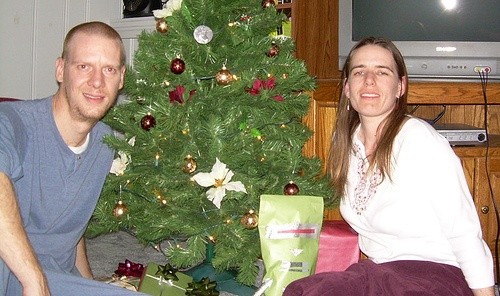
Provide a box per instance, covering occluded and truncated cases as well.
[301,79,500,284]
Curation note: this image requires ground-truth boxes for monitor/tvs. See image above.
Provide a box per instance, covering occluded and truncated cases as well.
[339,0,500,81]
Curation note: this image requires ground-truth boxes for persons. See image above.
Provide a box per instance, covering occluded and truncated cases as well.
[0,21,153,296]
[282,35,495,296]
